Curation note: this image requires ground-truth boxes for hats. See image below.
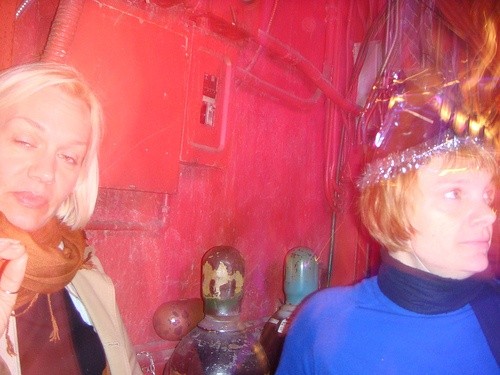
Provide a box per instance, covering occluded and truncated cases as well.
[354,67,483,191]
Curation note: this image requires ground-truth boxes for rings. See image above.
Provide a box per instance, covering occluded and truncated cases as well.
[0,286,21,294]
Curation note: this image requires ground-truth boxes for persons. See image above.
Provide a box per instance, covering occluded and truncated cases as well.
[271,96,500,375]
[0,61,144,375]
